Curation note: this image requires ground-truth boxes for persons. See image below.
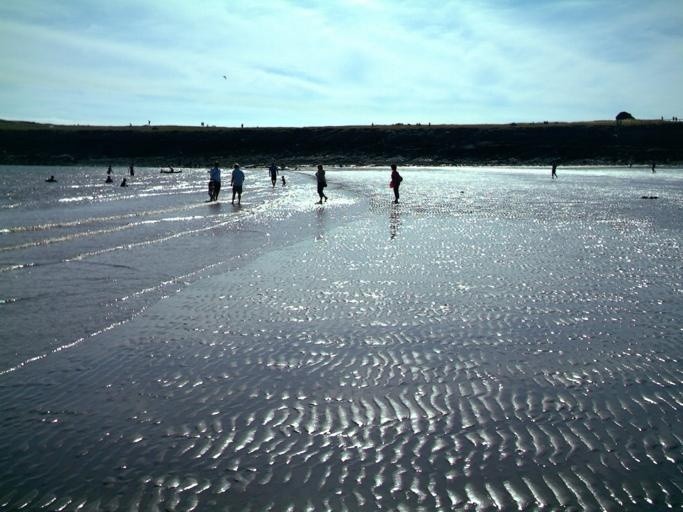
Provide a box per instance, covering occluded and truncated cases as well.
[651,161,656,172]
[269,163,278,187]
[391,164,402,203]
[551,160,558,178]
[231,163,244,205]
[316,165,328,204]
[392,203,400,239]
[208,162,221,200]
[45,161,134,187]
